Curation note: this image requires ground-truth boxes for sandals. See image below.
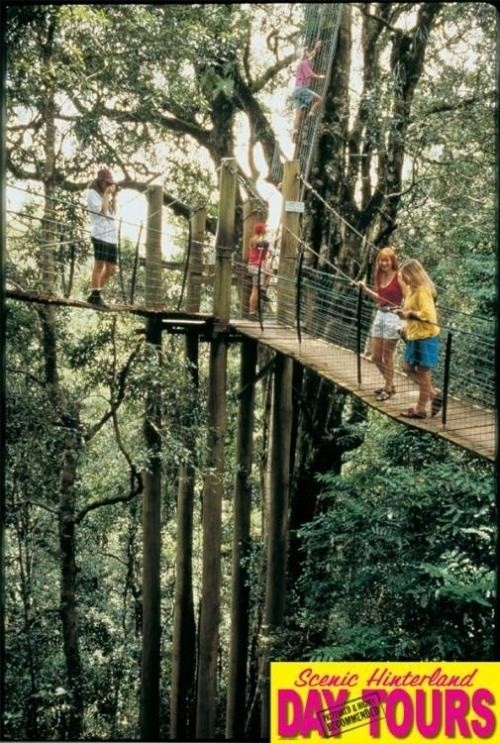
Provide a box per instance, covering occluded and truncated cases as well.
[373,386,397,403]
[401,406,428,420]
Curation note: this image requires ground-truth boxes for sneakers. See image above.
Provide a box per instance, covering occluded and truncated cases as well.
[430,392,444,418]
[86,290,107,307]
[247,311,260,323]
[259,288,271,302]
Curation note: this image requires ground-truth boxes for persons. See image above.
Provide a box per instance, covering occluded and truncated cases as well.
[247,219,273,323]
[392,258,444,417]
[290,39,327,144]
[355,246,410,398]
[81,167,123,308]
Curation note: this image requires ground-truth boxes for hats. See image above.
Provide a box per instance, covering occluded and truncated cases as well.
[254,223,269,234]
[97,168,116,185]
